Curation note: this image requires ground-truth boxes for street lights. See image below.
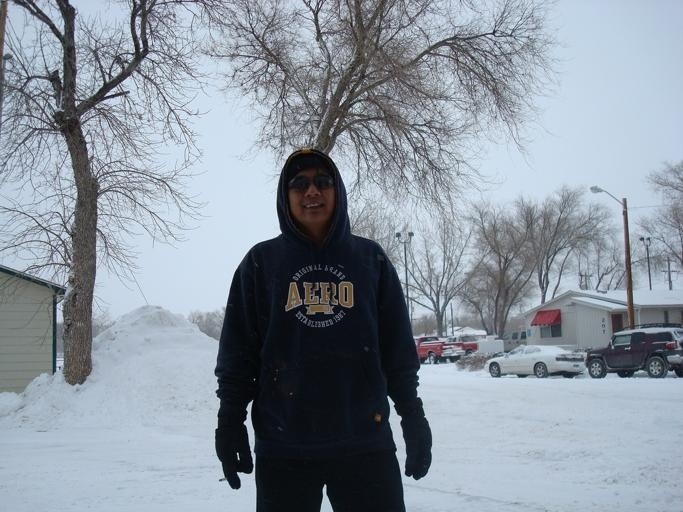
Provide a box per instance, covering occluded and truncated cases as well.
[393,231,413,315]
[589,185,635,330]
[637,235,652,291]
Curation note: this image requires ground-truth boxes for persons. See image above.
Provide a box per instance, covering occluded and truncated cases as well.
[214,147,432,512]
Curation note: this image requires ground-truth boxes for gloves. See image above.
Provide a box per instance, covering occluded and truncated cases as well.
[392,396,434,480]
[214,398,254,491]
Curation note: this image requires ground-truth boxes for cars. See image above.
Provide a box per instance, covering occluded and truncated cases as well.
[483,344,585,379]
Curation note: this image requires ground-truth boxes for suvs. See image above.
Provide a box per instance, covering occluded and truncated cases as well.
[585,325,682,379]
[414,335,488,364]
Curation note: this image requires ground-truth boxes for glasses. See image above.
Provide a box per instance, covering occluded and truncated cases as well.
[284,174,335,190]
